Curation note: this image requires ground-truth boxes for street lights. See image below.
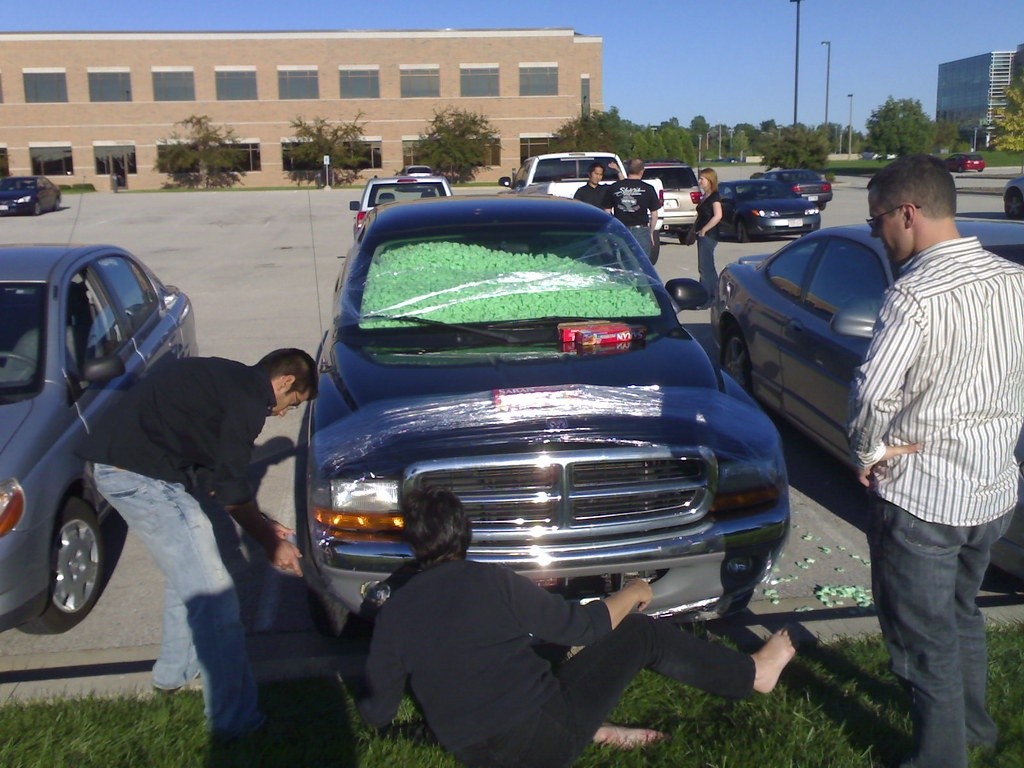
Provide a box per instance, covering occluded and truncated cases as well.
[848,94,853,158]
[790,0,802,126]
[820,41,831,125]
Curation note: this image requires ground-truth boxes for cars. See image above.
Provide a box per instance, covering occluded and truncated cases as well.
[713,179,822,243]
[742,168,833,210]
[395,165,433,178]
[709,217,1024,585]
[0,240,199,636]
[292,190,793,643]
[1002,174,1024,220]
[349,176,456,245]
[945,152,986,174]
[0,175,63,217]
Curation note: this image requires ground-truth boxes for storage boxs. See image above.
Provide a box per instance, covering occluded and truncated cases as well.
[492,384,582,406]
[556,321,648,346]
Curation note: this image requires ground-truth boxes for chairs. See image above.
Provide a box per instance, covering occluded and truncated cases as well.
[5,281,87,383]
[421,191,436,197]
[379,193,394,204]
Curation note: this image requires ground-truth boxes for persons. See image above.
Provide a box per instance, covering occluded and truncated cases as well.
[687,169,722,310]
[600,159,660,260]
[354,488,800,768]
[73,347,319,747]
[573,162,624,214]
[112,172,119,193]
[846,146,1024,768]
[14,180,25,190]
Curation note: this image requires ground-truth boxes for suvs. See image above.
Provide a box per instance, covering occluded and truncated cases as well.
[621,159,702,246]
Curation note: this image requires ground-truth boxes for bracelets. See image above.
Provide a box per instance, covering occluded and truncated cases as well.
[616,170,622,173]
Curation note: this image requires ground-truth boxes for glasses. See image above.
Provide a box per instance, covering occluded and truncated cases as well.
[865,204,920,229]
[287,392,298,410]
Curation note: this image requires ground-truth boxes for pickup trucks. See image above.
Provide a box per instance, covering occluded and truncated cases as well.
[496,151,665,266]
